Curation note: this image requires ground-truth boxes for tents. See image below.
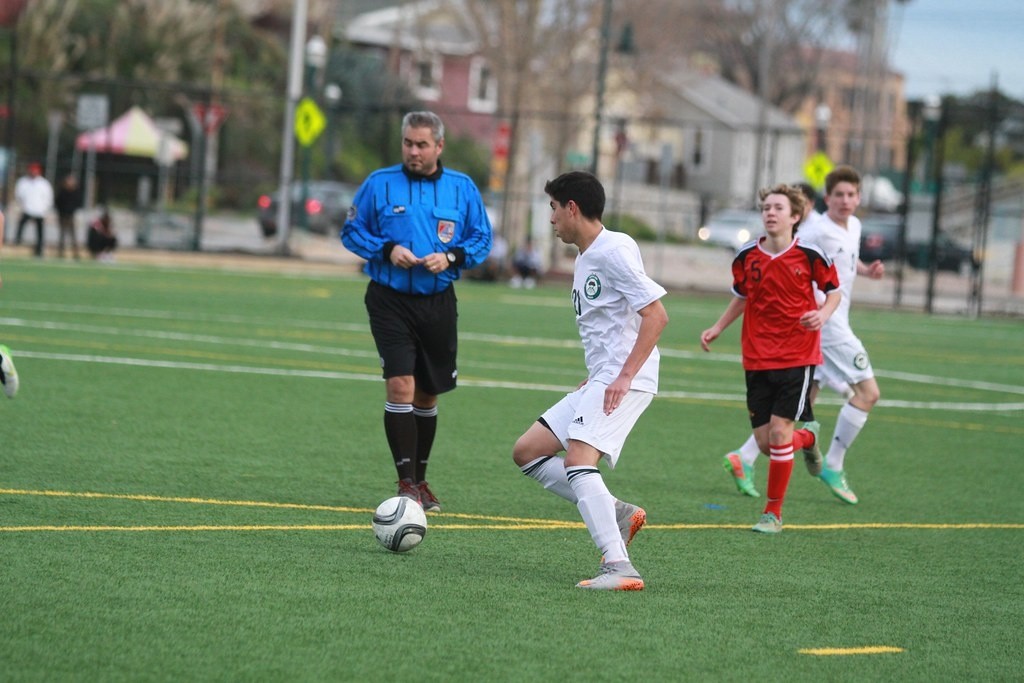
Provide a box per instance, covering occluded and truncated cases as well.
[74,104,189,210]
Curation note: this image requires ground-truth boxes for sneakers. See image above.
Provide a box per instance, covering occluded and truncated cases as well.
[802,420,823,477]
[751,513,784,533]
[600,502,646,564]
[722,449,760,497]
[0,345,19,400]
[398,480,422,508]
[575,562,645,591]
[417,482,441,512]
[816,456,857,503]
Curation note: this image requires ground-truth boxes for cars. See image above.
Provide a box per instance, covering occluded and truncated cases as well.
[859,220,981,281]
[258,181,353,238]
[699,202,765,251]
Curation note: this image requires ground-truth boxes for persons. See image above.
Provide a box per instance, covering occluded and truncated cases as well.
[700,182,841,535]
[340,113,493,510]
[723,163,884,504]
[0,213,18,397]
[13,162,54,256]
[88,211,117,264]
[479,231,544,288]
[54,176,81,257]
[512,170,668,589]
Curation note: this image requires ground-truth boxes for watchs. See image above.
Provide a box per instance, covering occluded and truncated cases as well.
[446,251,455,266]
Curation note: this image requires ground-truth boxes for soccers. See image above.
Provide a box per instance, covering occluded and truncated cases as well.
[371,497,428,552]
